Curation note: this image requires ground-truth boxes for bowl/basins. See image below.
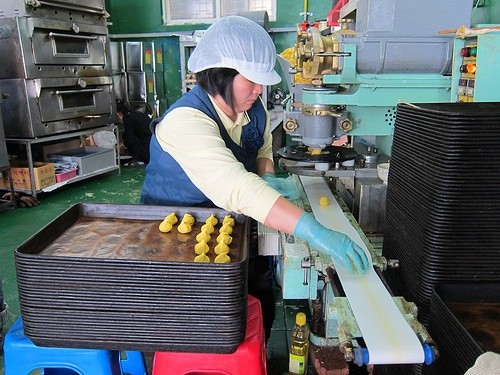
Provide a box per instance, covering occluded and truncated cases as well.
[377,163,390,183]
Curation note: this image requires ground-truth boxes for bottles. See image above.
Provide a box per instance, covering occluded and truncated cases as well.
[289,312,310,375]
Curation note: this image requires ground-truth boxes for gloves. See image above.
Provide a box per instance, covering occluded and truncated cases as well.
[293,212,370,276]
[262,172,298,199]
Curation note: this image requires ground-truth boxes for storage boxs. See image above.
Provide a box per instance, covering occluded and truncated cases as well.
[47,146,116,175]
[4,160,56,191]
[55,166,79,183]
[110,42,169,118]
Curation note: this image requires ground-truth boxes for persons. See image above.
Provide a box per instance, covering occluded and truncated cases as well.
[140,15,370,349]
[117,99,152,166]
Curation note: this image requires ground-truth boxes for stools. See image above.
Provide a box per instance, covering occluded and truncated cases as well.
[2,314,149,375]
[151,293,270,375]
